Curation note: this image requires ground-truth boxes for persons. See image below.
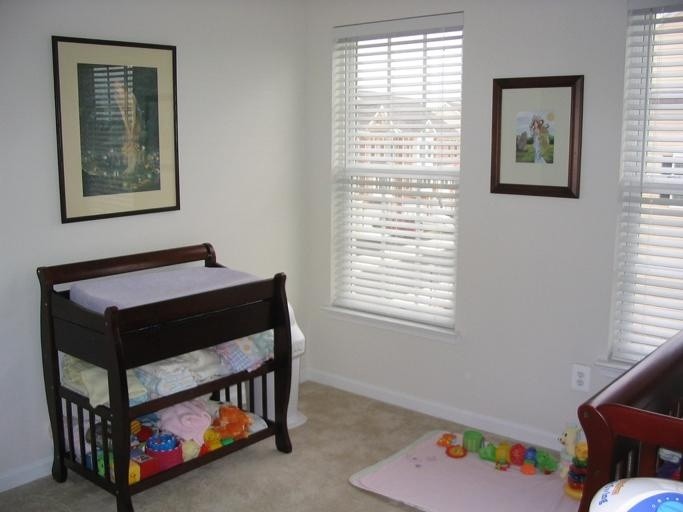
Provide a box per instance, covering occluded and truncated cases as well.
[530,120,546,164]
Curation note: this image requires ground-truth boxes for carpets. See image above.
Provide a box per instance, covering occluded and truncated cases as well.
[348,429,581,512]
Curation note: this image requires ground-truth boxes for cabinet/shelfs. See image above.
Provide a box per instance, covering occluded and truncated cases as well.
[36,242,291,511]
[577,327,683,512]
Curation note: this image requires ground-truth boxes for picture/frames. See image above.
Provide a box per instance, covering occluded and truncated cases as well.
[50,35,180,225]
[490,75,584,199]
